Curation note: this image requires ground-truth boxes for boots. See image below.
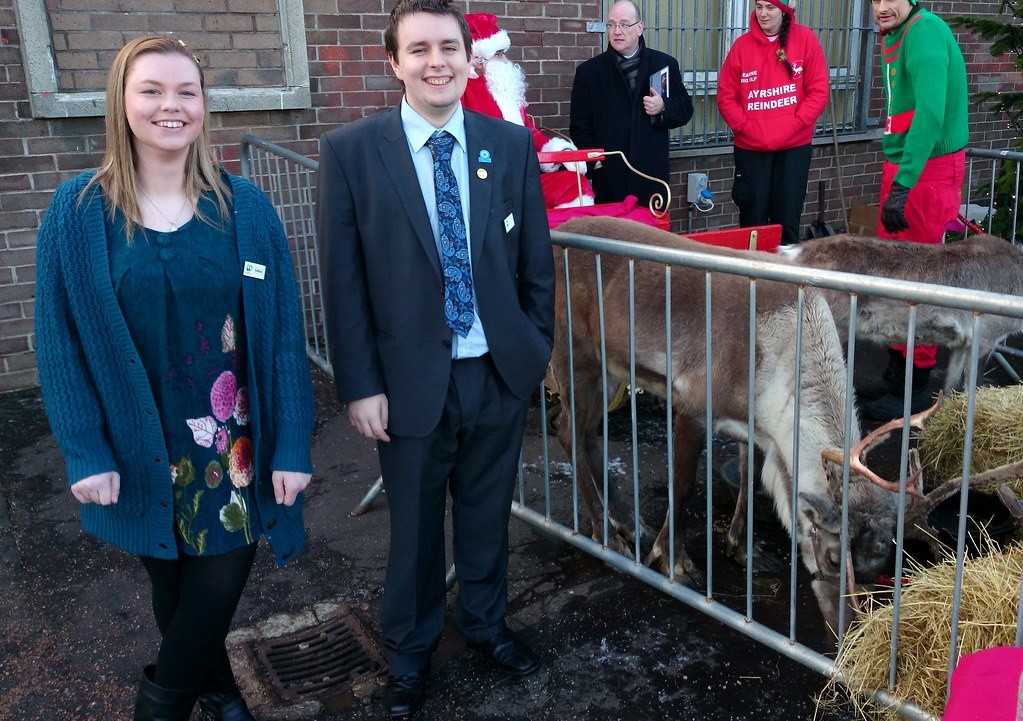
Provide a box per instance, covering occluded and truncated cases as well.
[198,657,256,721]
[133,664,202,721]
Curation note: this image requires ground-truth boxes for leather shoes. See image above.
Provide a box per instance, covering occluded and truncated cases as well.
[385,658,432,716]
[466,626,539,675]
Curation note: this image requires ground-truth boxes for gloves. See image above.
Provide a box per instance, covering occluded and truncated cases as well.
[881,181,910,235]
[562,149,588,175]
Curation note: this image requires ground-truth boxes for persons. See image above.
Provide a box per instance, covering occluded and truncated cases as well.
[716,0,831,248]
[871,0,970,398]
[33,34,316,721]
[319,0,562,714]
[460,13,595,210]
[568,0,695,209]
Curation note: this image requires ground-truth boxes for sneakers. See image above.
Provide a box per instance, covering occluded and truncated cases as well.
[886,355,903,384]
[897,367,930,395]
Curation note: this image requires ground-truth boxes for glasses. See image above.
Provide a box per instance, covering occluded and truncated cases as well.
[606,21,640,31]
[495,51,505,59]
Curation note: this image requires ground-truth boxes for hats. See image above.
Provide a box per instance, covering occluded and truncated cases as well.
[463,13,511,60]
[755,0,796,15]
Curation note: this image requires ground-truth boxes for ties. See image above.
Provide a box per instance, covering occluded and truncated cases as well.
[425,135,477,339]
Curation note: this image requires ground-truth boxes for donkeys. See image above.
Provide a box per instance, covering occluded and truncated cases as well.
[548,218,931,649]
[775,232,1023,399]
